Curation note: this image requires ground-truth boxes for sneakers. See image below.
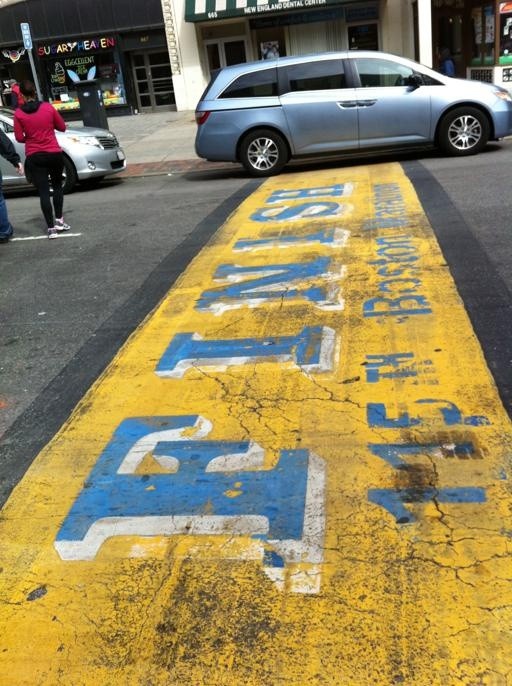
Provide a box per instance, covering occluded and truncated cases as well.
[48,216,71,239]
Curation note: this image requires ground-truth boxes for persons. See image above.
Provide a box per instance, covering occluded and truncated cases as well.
[262,43,279,60]
[0,79,71,243]
[439,46,456,77]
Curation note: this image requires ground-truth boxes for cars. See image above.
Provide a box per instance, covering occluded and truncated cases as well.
[0,103,128,196]
[188,48,511,172]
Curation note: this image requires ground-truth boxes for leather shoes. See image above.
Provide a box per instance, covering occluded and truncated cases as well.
[0,222,13,242]
